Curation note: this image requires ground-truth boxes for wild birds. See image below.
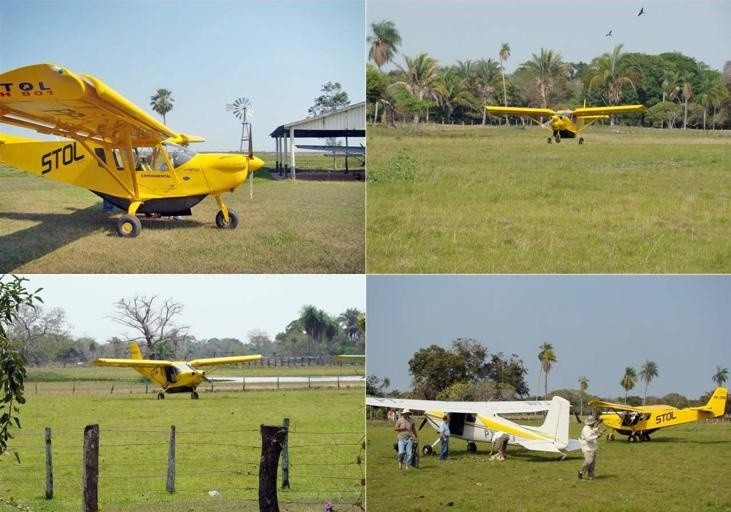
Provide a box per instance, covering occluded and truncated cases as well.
[638,8,646,16]
[605,30,612,36]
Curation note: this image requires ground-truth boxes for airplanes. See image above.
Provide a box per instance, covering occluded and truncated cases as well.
[482,102,646,145]
[293,142,365,167]
[365,395,582,462]
[0,60,266,239]
[585,386,728,444]
[89,341,264,401]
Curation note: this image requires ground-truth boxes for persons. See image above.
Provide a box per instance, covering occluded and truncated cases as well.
[624,411,651,442]
[489,430,511,461]
[387,407,403,426]
[437,414,453,461]
[394,408,420,472]
[578,414,604,480]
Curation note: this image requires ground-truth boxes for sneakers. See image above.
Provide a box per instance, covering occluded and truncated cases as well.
[578,469,594,480]
[398,464,410,472]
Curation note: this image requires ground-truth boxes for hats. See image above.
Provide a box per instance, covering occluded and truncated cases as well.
[585,415,597,425]
[400,407,413,416]
